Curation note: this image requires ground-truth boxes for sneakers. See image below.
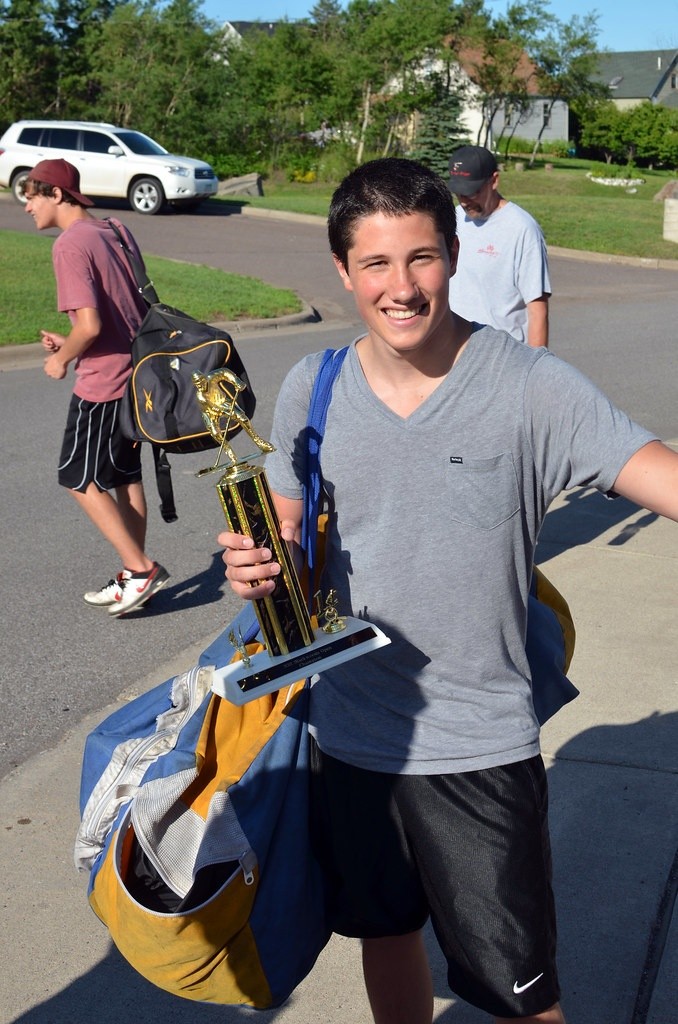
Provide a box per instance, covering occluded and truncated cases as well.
[83,568,135,606]
[107,561,171,618]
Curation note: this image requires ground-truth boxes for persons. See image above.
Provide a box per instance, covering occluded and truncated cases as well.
[18,159,170,615]
[218,157,678,1024]
[446,146,552,350]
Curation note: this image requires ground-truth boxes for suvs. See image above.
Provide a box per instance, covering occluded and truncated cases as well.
[0,120,218,215]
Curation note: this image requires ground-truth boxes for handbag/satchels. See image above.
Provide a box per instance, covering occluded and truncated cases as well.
[118,302,256,455]
[74,517,577,1013]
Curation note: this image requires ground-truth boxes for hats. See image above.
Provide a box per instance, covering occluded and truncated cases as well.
[444,144,498,196]
[29,157,95,206]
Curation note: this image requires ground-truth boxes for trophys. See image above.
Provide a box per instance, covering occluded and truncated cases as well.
[190,367,392,707]
